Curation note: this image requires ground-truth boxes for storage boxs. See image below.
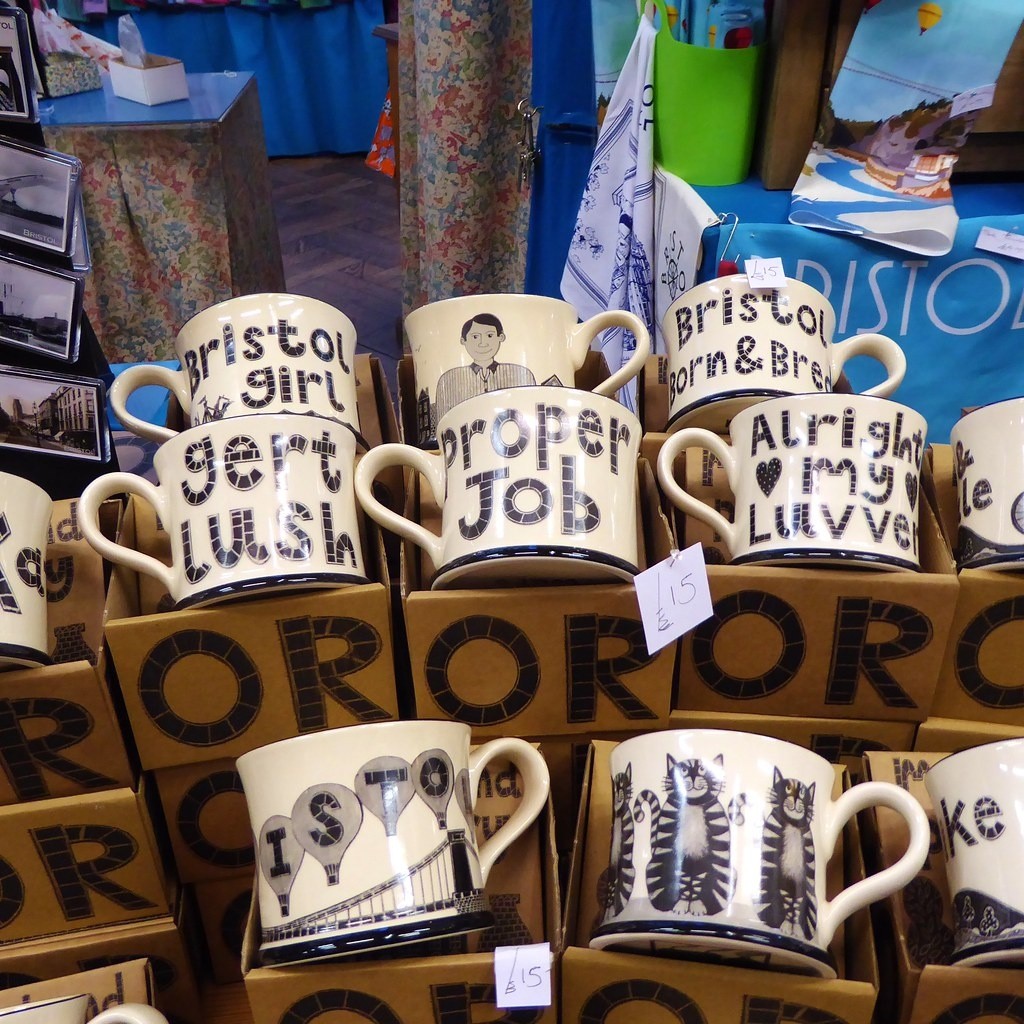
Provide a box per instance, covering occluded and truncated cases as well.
[0,352,1024,1023]
[106,51,188,106]
[39,52,104,100]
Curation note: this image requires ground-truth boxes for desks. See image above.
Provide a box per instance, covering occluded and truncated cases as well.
[652,165,1024,447]
[39,71,289,362]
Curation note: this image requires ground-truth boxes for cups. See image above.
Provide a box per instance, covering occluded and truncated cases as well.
[950,397,1024,574]
[663,274,907,434]
[77,414,371,610]
[1,472,54,667]
[923,737,1024,967]
[404,294,650,456]
[1,993,171,1024]
[110,293,371,453]
[354,385,642,591]
[236,720,550,968]
[657,394,928,572]
[588,729,930,979]
